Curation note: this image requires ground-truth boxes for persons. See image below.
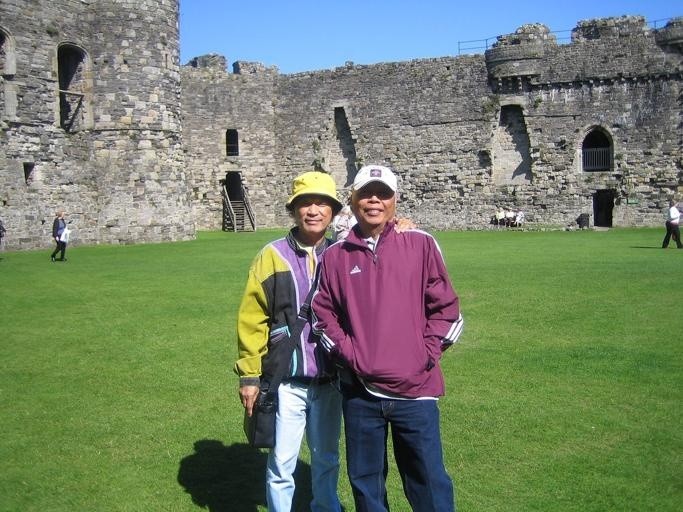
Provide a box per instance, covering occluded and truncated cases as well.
[506,208,515,227]
[496,207,506,227]
[0,220,5,247]
[513,208,526,227]
[330,204,355,242]
[308,161,465,511]
[231,169,420,510]
[661,200,683,249]
[50,209,68,262]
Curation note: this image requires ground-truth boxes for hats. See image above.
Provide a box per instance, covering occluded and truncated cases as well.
[285,172,343,215]
[353,165,397,191]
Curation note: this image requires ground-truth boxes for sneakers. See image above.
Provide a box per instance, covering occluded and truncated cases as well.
[61,259,66,261]
[52,255,55,261]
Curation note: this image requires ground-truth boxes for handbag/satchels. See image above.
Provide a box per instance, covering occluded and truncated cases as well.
[243,383,279,449]
[60,229,69,243]
